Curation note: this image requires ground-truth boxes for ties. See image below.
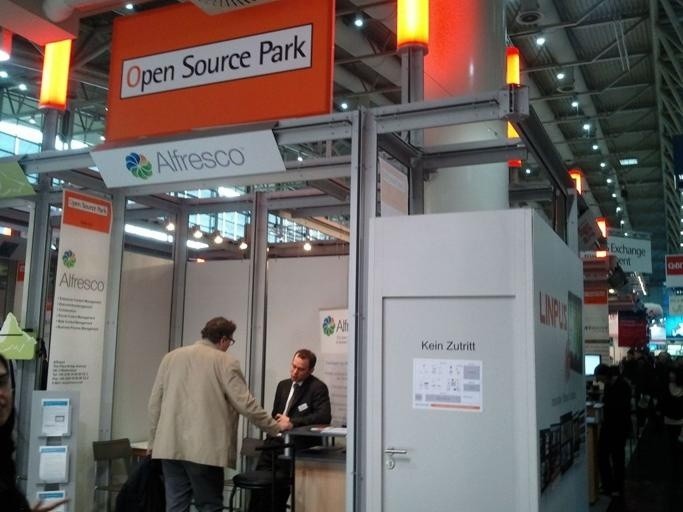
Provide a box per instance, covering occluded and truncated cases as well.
[287,384,300,415]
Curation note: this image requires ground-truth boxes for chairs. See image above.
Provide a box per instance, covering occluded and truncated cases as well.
[229,438,288,511]
[94,439,133,512]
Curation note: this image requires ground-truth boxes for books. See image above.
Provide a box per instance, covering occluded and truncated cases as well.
[320,426,346,436]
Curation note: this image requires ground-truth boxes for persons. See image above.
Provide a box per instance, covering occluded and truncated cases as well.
[0,352,72,512]
[592,345,682,504]
[246,347,332,512]
[145,315,293,512]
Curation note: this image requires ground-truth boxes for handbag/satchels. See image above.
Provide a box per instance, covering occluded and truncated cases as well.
[115,455,165,512]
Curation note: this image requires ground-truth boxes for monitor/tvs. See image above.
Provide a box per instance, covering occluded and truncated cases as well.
[584,353,601,382]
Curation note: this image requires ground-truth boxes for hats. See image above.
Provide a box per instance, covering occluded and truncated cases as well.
[594,363,609,381]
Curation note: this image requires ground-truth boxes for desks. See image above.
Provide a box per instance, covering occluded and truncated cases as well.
[130,442,151,468]
[282,424,346,511]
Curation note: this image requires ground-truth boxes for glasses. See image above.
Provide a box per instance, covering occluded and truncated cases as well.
[228,336,236,346]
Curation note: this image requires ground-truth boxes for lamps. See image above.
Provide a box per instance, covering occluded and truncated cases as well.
[38,38,72,114]
[397,0,428,55]
[163,217,311,252]
[506,47,520,86]
[507,122,521,167]
[596,218,606,258]
[568,168,583,195]
